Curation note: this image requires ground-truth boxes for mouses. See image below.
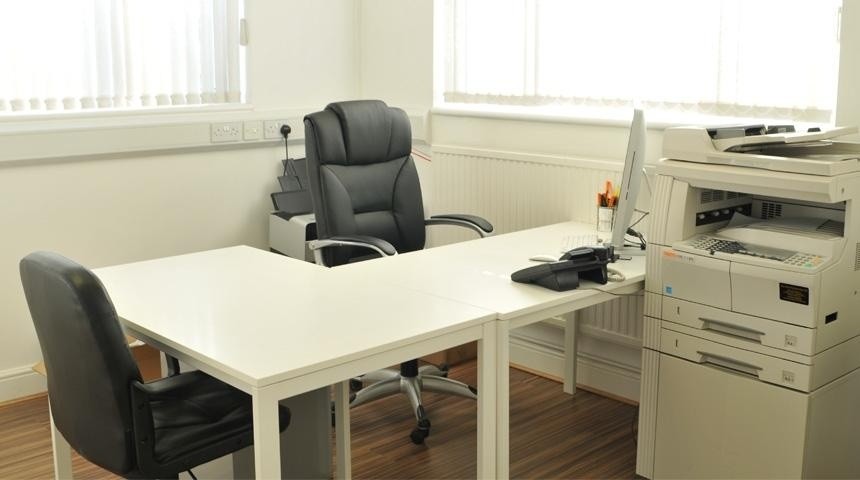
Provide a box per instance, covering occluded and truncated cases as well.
[529,253,557,263]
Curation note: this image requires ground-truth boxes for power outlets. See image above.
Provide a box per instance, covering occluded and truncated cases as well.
[209,117,299,143]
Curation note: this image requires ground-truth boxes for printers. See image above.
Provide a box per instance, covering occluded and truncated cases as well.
[637,115,859,480]
[268,190,317,262]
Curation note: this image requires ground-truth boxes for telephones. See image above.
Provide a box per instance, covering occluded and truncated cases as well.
[511,246,611,292]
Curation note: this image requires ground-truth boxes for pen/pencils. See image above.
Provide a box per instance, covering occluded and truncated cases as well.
[597,182,621,208]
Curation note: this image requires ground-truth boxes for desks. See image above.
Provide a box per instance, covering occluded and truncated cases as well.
[88,219,648,480]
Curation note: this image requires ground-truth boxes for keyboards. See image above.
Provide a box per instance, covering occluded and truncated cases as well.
[560,231,599,253]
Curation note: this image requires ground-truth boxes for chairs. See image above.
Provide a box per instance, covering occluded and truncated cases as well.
[301,97,497,445]
[18,248,293,480]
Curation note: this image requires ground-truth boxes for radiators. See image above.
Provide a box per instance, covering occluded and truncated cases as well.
[428,139,656,348]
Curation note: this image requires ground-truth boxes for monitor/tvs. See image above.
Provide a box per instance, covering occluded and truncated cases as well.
[603,109,646,258]
